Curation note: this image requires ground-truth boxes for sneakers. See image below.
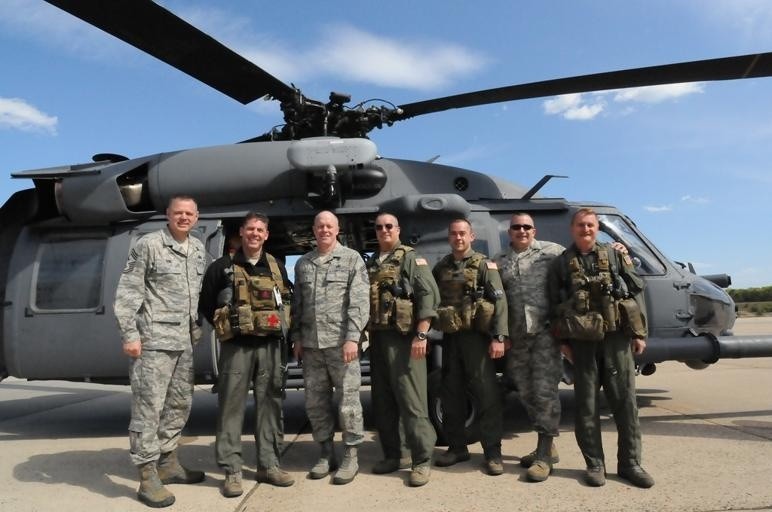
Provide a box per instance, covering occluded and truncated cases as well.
[409,465,430,487]
[585,464,607,486]
[223,471,243,497]
[256,465,295,487]
[484,447,504,475]
[372,457,400,474]
[617,462,654,488]
[434,446,470,467]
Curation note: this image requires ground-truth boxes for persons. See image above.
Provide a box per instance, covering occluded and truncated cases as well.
[432,218,510,475]
[292,212,372,483]
[493,211,629,481]
[110,194,216,508]
[361,215,441,485]
[546,208,655,488]
[198,212,296,497]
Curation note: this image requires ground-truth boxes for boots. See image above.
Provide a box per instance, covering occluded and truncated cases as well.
[333,445,359,484]
[156,448,205,484]
[520,440,559,468]
[526,433,553,481]
[137,460,175,508]
[310,439,335,479]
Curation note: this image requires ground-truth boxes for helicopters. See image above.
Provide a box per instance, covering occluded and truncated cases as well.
[0,1,772,443]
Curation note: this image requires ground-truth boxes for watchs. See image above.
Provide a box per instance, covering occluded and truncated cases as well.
[413,330,427,339]
[492,334,506,342]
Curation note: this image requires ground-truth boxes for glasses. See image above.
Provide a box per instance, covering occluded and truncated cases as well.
[510,225,534,230]
[375,224,401,230]
